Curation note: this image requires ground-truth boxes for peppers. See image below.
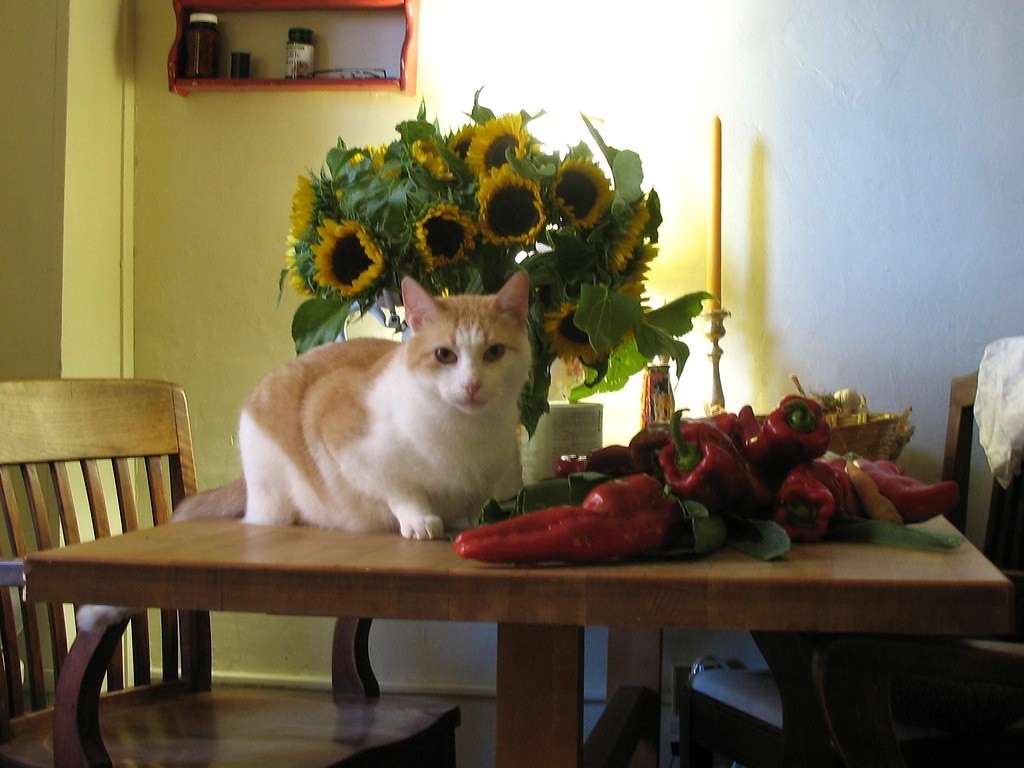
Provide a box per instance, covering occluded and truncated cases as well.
[454,387,963,562]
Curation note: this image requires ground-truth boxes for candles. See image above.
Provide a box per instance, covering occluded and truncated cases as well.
[706,112,724,314]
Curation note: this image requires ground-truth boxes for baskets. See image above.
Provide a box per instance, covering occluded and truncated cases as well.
[756,399,897,460]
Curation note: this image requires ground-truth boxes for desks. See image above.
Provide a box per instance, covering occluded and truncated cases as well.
[22,502,1018,768]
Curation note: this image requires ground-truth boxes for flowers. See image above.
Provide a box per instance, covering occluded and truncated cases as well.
[270,86,719,409]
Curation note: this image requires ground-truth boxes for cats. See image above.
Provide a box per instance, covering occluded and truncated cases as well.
[76,266,532,630]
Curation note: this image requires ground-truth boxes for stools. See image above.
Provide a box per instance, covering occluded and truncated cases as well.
[689,668,786,768]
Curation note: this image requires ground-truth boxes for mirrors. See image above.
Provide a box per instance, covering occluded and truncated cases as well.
[659,628,786,768]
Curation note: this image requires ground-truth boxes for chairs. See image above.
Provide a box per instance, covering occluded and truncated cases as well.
[0,376,462,768]
[809,335,1024,768]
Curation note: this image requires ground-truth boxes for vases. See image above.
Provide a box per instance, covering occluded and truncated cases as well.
[514,403,604,485]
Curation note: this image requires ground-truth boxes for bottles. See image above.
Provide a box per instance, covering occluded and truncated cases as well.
[641,362,676,431]
[284,27,316,79]
[230,51,251,78]
[183,12,224,80]
[517,403,603,487]
